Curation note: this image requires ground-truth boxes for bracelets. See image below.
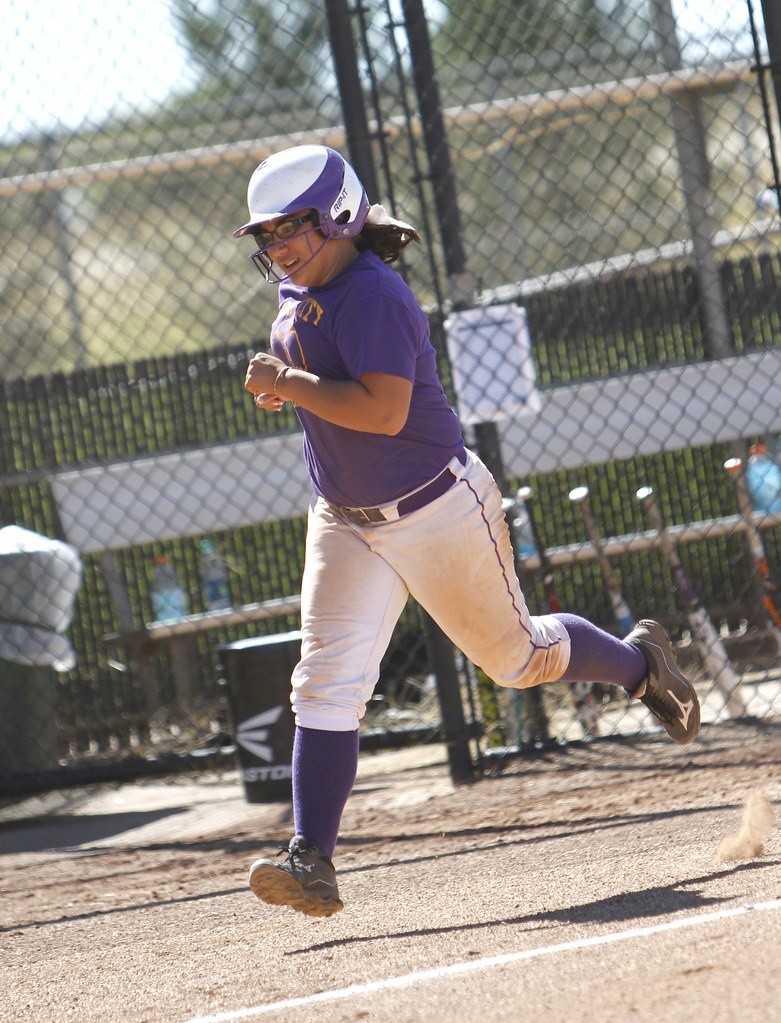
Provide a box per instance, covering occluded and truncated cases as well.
[274,366,291,396]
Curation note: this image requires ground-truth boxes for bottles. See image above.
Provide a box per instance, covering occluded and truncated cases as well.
[149,556,187,622]
[746,442,781,515]
[198,538,231,611]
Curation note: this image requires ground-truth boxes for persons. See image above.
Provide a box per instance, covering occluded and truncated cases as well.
[232,144,699,917]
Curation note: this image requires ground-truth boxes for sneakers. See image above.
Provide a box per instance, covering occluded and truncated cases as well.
[250,834,343,918]
[623,618,700,743]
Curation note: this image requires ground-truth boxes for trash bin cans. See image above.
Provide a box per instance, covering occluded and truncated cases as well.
[215,630,304,804]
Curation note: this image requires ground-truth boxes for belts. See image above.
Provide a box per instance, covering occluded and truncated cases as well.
[326,446,468,524]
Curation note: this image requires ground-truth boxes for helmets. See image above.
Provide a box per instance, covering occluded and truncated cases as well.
[233,143,371,239]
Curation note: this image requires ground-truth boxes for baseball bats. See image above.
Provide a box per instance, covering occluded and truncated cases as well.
[636,486,752,723]
[500,496,518,569]
[567,484,637,633]
[517,484,601,741]
[724,459,779,629]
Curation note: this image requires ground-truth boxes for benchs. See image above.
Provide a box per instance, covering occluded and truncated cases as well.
[50,350,781,727]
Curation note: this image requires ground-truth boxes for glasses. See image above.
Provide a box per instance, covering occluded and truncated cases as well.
[255,213,316,249]
